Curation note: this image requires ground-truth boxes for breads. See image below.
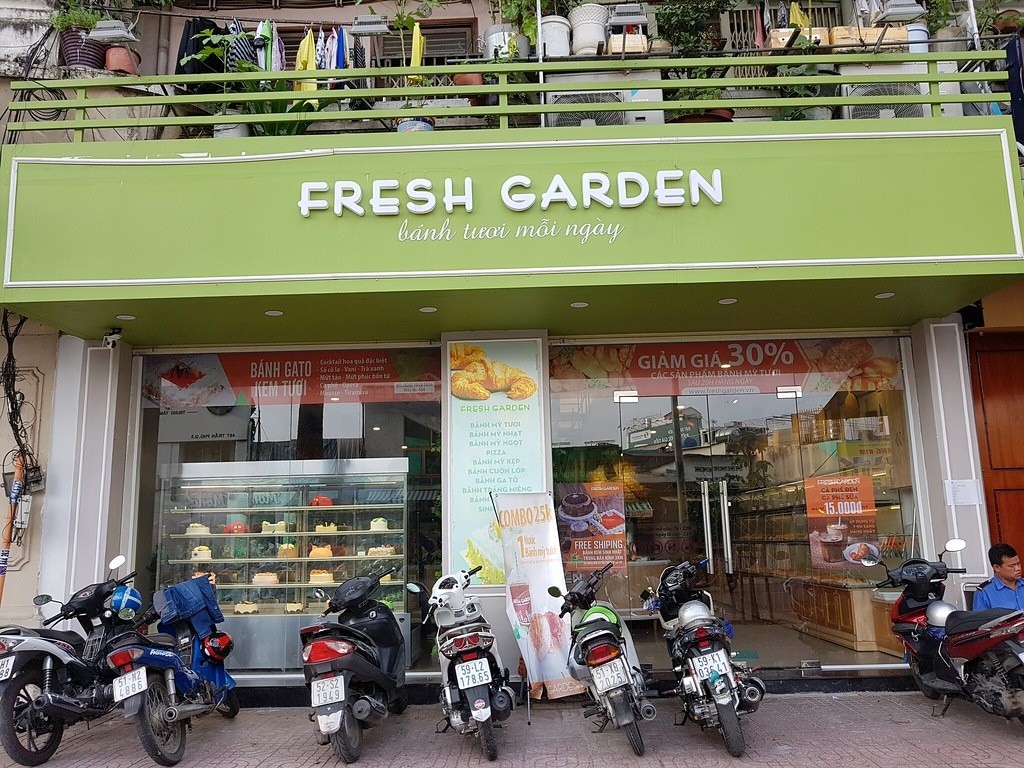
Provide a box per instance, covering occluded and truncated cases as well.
[798,336,899,392]
[751,566,865,584]
[449,343,537,400]
[548,345,636,392]
[851,544,870,561]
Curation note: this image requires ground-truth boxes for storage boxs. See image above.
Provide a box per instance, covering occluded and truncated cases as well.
[764,28,829,54]
[829,26,910,54]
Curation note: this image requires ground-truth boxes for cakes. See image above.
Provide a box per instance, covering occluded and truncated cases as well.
[184,516,395,615]
[561,492,625,536]
[141,358,222,411]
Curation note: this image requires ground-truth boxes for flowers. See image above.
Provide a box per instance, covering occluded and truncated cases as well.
[625,25,640,35]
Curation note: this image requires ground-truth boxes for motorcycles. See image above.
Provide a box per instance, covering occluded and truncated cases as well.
[101,573,239,767]
[209,559,402,605]
[300,562,408,765]
[783,574,851,657]
[860,539,1024,728]
[0,556,146,766]
[404,566,517,762]
[638,554,768,759]
[548,562,657,757]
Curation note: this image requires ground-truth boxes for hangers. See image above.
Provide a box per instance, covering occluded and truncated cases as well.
[301,19,309,41]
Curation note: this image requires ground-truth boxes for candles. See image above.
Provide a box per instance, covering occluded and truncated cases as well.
[453,59,484,85]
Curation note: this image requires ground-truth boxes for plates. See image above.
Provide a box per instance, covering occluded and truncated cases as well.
[844,542,879,563]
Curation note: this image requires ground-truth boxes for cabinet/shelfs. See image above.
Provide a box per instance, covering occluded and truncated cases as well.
[154,457,424,673]
[869,598,905,659]
[729,464,909,653]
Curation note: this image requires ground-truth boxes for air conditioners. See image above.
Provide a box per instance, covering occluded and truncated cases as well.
[544,69,665,127]
[837,60,964,119]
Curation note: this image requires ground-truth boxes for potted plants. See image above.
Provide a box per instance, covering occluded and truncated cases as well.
[655,0,736,122]
[975,0,1024,35]
[483,0,530,58]
[772,33,836,121]
[367,0,446,132]
[927,0,967,52]
[50,0,175,69]
[105,45,142,75]
[502,0,571,57]
[563,0,609,56]
[179,28,275,138]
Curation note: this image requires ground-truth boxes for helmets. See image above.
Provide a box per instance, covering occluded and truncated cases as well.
[110,586,142,613]
[199,632,234,664]
[925,601,958,629]
[677,601,712,629]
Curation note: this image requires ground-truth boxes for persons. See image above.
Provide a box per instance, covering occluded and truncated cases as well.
[972,543,1024,612]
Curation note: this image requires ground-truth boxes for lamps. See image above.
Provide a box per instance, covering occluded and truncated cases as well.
[873,0,929,22]
[347,15,393,37]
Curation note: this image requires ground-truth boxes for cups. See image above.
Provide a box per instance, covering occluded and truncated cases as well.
[819,534,844,563]
[827,524,848,545]
[510,581,533,625]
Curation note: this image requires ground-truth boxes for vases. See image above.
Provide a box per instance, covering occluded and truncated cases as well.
[647,36,674,52]
[608,34,648,54]
[903,23,930,53]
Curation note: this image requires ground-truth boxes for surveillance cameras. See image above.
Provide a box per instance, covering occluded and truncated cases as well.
[105,329,121,349]
